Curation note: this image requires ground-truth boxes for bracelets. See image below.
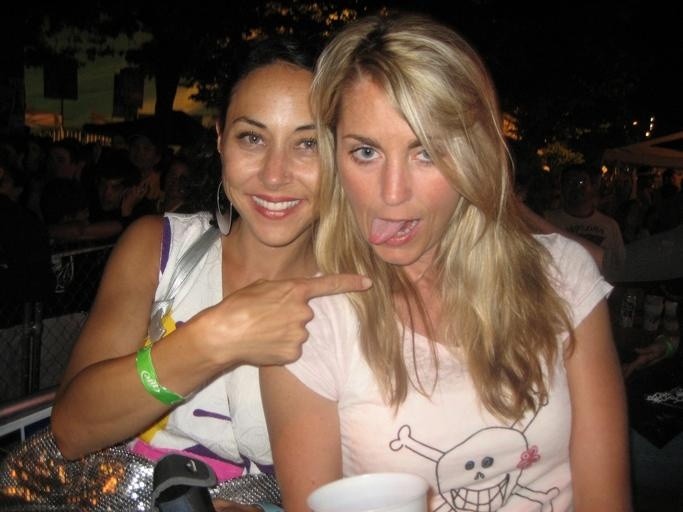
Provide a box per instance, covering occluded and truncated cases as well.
[134,338,197,408]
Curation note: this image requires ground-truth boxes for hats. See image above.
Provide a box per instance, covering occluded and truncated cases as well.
[556,163,598,184]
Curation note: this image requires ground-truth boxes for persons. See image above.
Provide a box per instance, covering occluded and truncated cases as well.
[47,29,373,512]
[255,11,636,510]
[0,119,233,321]
[500,132,682,384]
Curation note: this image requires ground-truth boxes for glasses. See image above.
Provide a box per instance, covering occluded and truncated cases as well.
[563,177,587,186]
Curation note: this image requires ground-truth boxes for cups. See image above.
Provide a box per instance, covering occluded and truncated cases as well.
[306,470,430,512]
